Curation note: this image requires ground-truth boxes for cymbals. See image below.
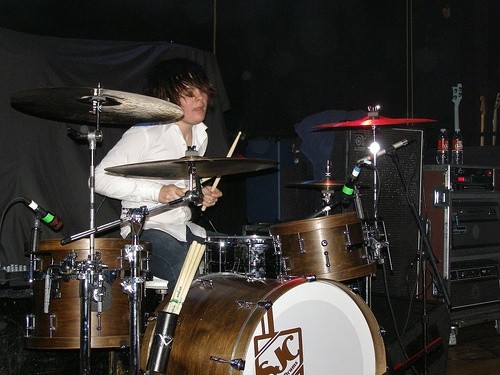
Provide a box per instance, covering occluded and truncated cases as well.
[10,87,184,126]
[310,115,441,129]
[104,155,281,179]
[286,179,354,188]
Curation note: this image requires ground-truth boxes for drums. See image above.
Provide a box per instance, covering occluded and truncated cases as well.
[139,274,387,375]
[200,234,277,278]
[269,211,379,282]
[23,237,152,349]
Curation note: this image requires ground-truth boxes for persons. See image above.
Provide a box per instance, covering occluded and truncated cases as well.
[88,57,223,300]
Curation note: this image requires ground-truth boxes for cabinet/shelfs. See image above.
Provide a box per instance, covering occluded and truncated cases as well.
[423,164,500,345]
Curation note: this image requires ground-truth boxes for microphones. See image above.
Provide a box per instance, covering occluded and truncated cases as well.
[338,163,362,205]
[23,198,64,233]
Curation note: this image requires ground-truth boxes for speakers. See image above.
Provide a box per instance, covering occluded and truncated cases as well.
[364,293,449,375]
[276,127,425,344]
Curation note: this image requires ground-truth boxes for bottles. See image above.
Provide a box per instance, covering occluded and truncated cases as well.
[436,128,449,165]
[451,129,464,165]
